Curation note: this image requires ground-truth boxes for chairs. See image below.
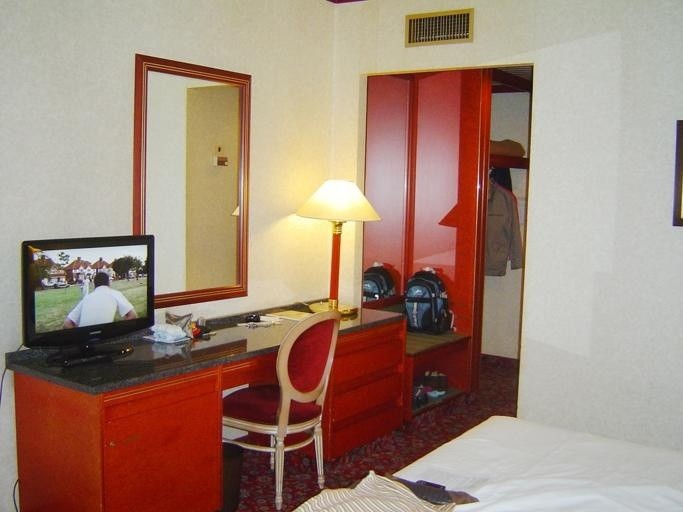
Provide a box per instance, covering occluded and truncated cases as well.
[220,309,343,511]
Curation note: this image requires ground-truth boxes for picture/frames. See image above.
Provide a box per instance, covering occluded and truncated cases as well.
[672,119,682,227]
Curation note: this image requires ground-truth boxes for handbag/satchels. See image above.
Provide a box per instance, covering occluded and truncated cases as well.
[165,312,194,340]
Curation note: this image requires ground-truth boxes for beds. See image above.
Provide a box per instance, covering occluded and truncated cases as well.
[389,416,683,511]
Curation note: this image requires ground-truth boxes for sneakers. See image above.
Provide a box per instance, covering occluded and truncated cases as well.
[413,369,448,408]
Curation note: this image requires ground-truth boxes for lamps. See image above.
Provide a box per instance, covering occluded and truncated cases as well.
[296,180,380,316]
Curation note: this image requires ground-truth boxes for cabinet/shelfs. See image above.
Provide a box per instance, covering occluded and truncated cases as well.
[10,371,225,511]
[325,315,473,465]
[417,62,534,400]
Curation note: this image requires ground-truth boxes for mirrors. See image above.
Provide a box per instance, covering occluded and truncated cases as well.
[355,72,418,310]
[133,53,252,308]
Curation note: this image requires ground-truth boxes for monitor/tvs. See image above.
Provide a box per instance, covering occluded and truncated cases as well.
[21,234,155,368]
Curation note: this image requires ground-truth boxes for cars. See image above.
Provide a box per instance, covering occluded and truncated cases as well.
[44,278,67,288]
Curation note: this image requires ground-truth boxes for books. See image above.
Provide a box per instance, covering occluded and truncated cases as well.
[265,309,313,321]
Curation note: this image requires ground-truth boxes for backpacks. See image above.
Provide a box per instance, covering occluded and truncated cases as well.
[360,261,396,302]
[403,265,456,336]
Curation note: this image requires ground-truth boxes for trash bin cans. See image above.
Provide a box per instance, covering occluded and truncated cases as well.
[221,442,243,512]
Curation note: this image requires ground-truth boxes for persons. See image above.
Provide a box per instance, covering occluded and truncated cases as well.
[81,275,91,297]
[62,271,137,329]
